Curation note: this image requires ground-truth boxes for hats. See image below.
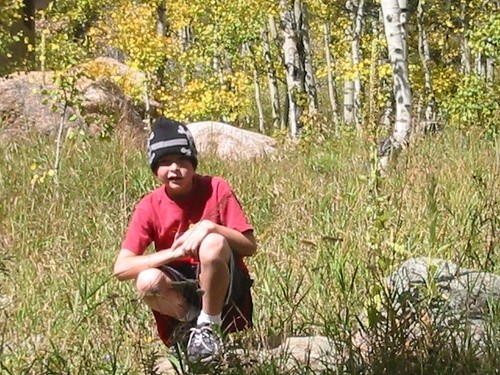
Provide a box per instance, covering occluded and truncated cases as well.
[146,117,198,172]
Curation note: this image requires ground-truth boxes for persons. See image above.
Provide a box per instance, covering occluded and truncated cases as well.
[114,117,256,359]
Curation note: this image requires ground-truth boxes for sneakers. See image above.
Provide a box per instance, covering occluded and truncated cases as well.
[187,322,224,363]
